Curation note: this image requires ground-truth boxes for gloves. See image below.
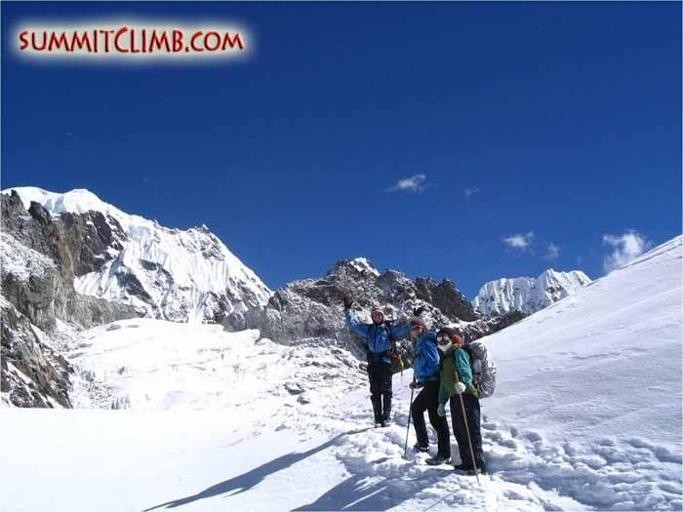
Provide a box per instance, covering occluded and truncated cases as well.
[343,294,354,308]
[455,382,466,394]
[414,307,423,316]
[437,403,446,417]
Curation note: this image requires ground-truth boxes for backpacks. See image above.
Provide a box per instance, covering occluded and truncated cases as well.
[462,342,496,398]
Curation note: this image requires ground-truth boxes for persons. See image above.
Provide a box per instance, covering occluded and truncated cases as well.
[435,326,488,473]
[343,294,424,427]
[408,319,453,465]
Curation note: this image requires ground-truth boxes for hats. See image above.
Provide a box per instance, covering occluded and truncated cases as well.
[436,328,453,342]
[409,319,425,329]
[371,305,384,318]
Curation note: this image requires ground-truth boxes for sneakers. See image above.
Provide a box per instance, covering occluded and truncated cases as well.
[455,464,481,474]
[416,443,430,451]
[426,455,452,465]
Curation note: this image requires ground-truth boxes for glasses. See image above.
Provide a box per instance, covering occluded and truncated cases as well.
[437,336,449,342]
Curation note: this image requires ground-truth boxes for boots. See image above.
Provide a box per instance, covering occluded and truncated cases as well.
[375,410,390,427]
